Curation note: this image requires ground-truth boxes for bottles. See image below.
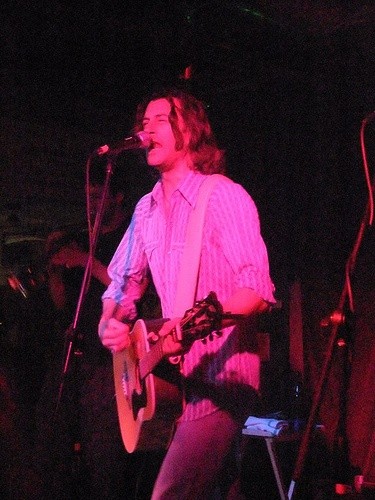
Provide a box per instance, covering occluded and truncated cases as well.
[289,371,305,436]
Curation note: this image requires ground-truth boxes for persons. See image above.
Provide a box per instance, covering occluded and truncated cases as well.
[96,90,276,500]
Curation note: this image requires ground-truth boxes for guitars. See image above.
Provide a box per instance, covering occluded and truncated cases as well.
[113,293,223,455]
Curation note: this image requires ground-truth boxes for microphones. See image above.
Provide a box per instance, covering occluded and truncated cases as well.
[96,130,151,156]
